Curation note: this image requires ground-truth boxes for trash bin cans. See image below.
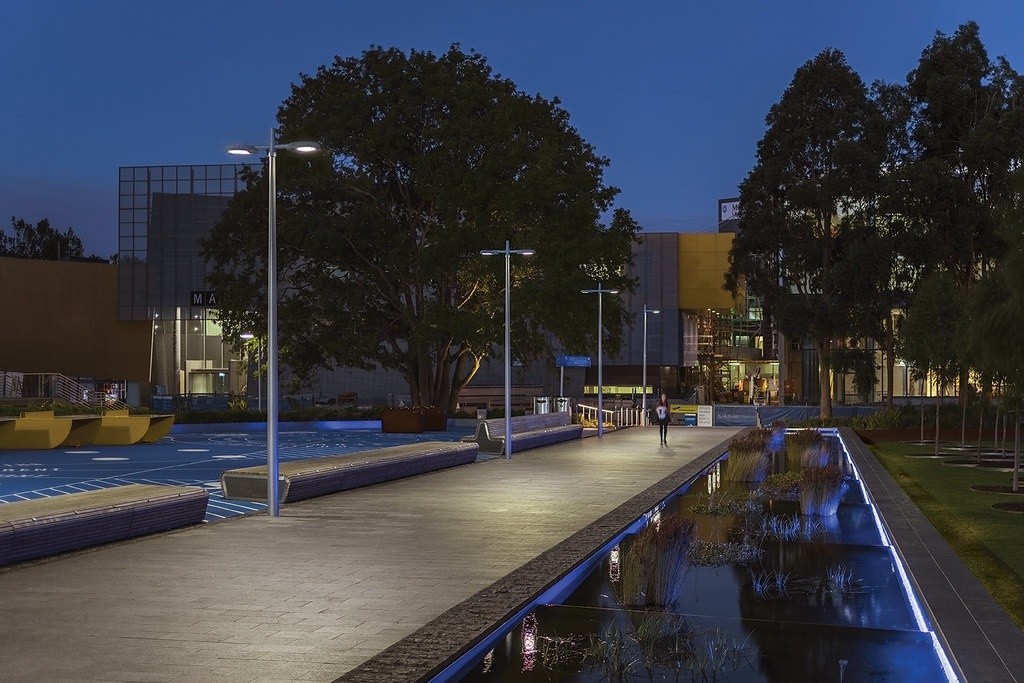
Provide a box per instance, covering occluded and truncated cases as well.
[532,396,552,415]
[554,397,572,423]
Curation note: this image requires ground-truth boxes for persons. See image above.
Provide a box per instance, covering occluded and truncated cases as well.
[655,393,674,446]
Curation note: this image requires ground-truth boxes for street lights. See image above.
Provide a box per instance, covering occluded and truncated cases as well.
[641,304,661,429]
[579,282,620,438]
[225,128,323,517]
[479,240,536,461]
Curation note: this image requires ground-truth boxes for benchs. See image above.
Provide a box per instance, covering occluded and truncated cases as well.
[461,412,584,455]
[0,483,210,568]
[221,441,479,504]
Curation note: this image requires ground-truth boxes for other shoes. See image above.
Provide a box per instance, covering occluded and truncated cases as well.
[665,442,668,446]
[660,442,663,445]
